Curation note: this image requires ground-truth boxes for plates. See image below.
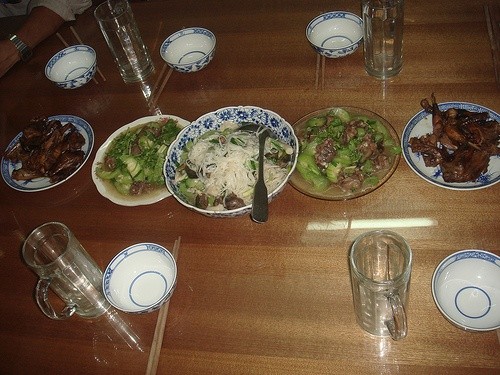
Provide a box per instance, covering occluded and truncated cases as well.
[286,106,401,200]
[0,115,94,192]
[91,115,192,207]
[401,101,500,190]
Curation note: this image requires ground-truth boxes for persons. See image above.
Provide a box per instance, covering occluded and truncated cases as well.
[0,0,92,78]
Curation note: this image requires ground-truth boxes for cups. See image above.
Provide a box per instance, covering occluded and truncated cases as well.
[359,0,404,79]
[94,0,154,83]
[349,230,413,342]
[21,221,112,321]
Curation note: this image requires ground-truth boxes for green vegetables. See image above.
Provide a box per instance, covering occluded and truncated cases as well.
[95,119,186,197]
[175,128,290,207]
[294,108,402,191]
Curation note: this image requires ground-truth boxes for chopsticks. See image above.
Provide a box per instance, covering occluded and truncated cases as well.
[313,11,329,90]
[56,27,107,84]
[144,236,182,375]
[145,64,173,112]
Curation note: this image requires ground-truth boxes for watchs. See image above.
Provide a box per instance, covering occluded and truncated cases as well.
[9,33,32,61]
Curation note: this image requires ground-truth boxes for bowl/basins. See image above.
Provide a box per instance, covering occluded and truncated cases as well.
[162,106,300,219]
[44,44,97,89]
[305,11,366,59]
[430,250,500,334]
[102,242,178,315]
[160,27,217,73]
